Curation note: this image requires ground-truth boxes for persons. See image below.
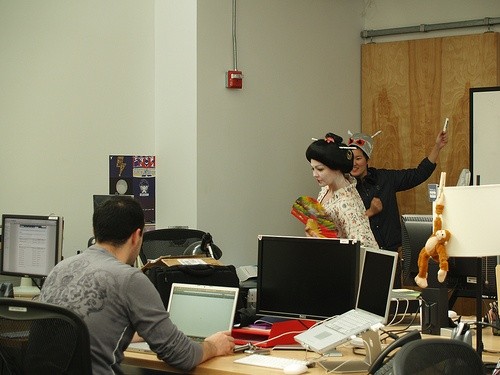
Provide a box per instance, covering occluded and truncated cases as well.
[30,196,235,375]
[346,130,448,315]
[290,133,379,250]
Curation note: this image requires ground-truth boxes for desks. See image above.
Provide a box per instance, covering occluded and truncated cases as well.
[117,312,500,375]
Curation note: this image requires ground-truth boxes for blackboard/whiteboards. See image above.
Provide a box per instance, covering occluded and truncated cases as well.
[468,86,500,185]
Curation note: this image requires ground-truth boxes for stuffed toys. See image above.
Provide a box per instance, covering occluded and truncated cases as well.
[413,194,450,288]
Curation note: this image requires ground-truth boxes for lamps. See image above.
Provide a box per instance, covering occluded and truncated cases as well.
[433,183,500,360]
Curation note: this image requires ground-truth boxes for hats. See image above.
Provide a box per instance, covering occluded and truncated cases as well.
[347,129,382,159]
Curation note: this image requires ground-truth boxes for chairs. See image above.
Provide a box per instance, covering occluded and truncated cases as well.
[393,339,491,375]
[0,298,93,375]
[139,229,221,265]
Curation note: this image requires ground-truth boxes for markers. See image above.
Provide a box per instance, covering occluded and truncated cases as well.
[442,117,449,135]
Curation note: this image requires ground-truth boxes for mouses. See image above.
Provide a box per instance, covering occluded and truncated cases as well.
[283,360,308,375]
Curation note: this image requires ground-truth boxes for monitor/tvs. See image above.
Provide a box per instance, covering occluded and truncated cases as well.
[400,214,498,328]
[255,235,360,321]
[0,214,64,289]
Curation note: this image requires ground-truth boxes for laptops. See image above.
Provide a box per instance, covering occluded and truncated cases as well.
[294,248,398,355]
[126,283,239,355]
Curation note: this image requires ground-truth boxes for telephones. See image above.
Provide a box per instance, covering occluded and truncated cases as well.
[368,328,424,375]
